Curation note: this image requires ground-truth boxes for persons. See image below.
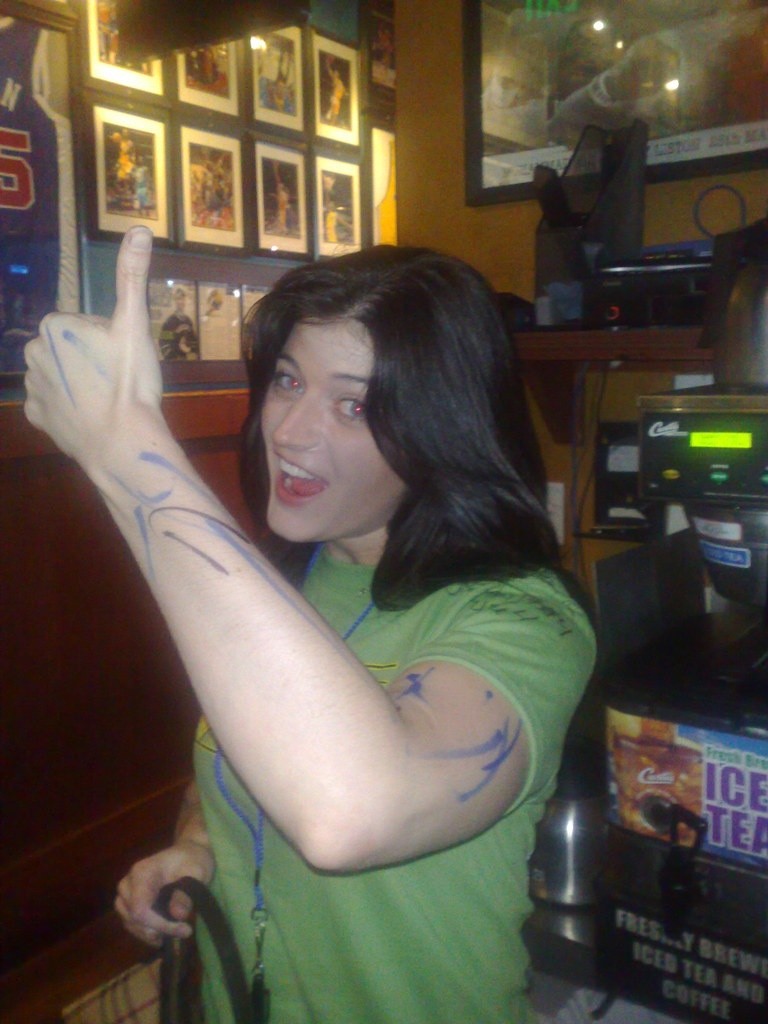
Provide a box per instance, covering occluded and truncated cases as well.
[324,182,352,242]
[22,226,597,1024]
[192,146,231,218]
[107,128,151,216]
[261,73,292,113]
[268,163,294,234]
[323,59,349,127]
[159,289,196,360]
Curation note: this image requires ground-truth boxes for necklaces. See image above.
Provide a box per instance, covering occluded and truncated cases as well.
[214,544,376,1024]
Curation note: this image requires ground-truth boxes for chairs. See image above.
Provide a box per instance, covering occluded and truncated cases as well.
[0,904,168,1024]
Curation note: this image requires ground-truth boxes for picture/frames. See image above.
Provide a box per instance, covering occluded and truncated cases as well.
[459,0,767,208]
[0,0,398,386]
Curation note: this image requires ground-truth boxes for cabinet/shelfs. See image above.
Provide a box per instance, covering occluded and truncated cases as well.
[512,323,729,594]
